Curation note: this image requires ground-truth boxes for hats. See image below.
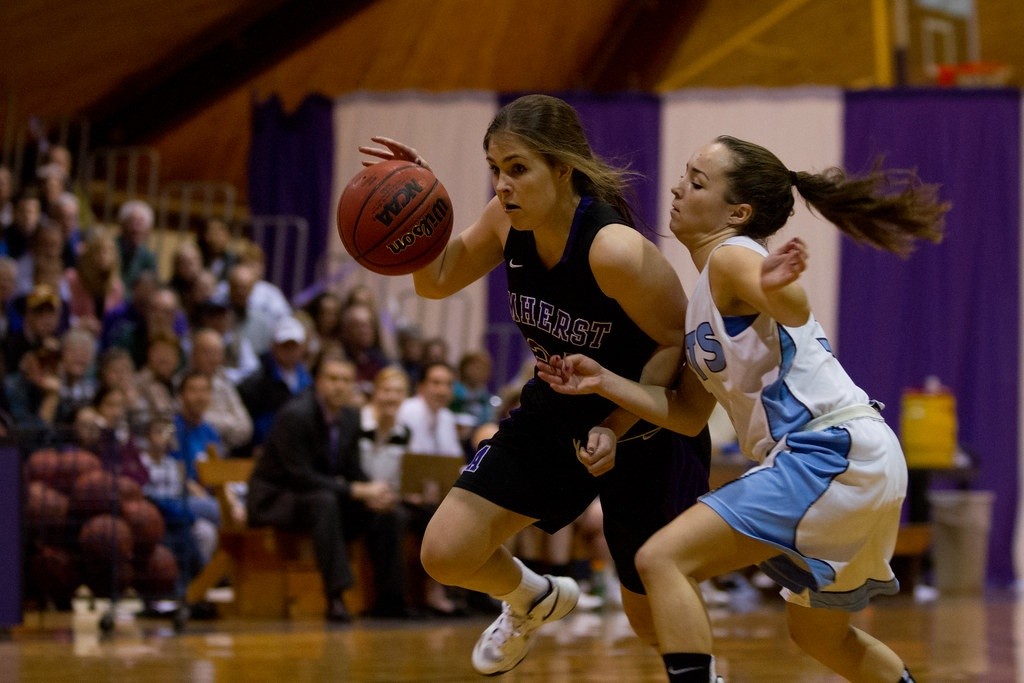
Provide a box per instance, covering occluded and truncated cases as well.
[274,315,309,347]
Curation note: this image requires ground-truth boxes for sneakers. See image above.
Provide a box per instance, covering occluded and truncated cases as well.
[471,574,580,677]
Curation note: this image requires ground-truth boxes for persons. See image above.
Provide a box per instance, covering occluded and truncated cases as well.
[358,93,715,682]
[0,143,627,631]
[536,133,953,683]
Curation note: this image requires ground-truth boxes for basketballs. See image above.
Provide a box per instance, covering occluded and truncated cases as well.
[335,158,456,277]
[18,445,179,610]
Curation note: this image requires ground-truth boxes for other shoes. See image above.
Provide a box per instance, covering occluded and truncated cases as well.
[49,564,785,624]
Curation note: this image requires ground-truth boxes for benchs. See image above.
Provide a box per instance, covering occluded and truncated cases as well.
[187,446,464,623]
